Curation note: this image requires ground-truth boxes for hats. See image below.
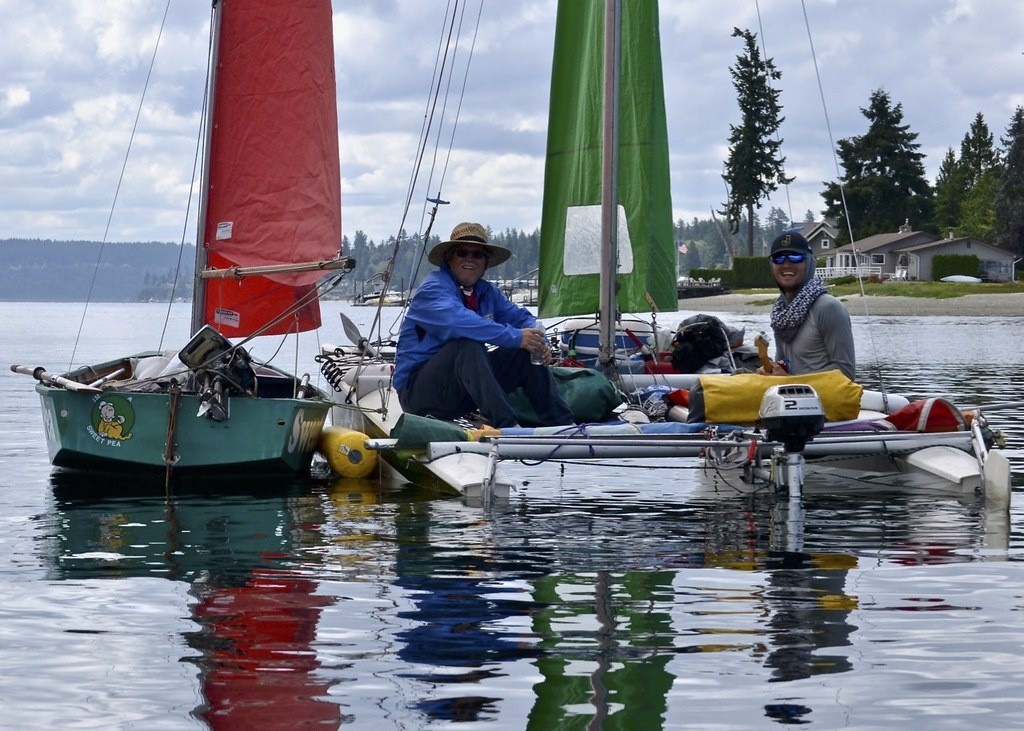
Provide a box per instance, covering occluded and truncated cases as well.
[428,221,512,270]
[771,229,808,255]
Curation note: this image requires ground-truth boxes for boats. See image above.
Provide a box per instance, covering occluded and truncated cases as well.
[350,271,413,306]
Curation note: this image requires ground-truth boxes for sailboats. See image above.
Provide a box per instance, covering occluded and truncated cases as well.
[318,0,1004,504]
[31,0,342,470]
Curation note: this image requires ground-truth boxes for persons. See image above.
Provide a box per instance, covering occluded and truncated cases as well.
[732,231,855,381]
[392,222,580,429]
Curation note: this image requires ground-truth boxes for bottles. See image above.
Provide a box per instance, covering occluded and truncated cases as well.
[530,319,546,365]
[630,385,678,396]
[549,335,562,359]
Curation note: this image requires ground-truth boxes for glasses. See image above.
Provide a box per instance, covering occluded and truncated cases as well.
[771,251,805,264]
[456,247,485,259]
